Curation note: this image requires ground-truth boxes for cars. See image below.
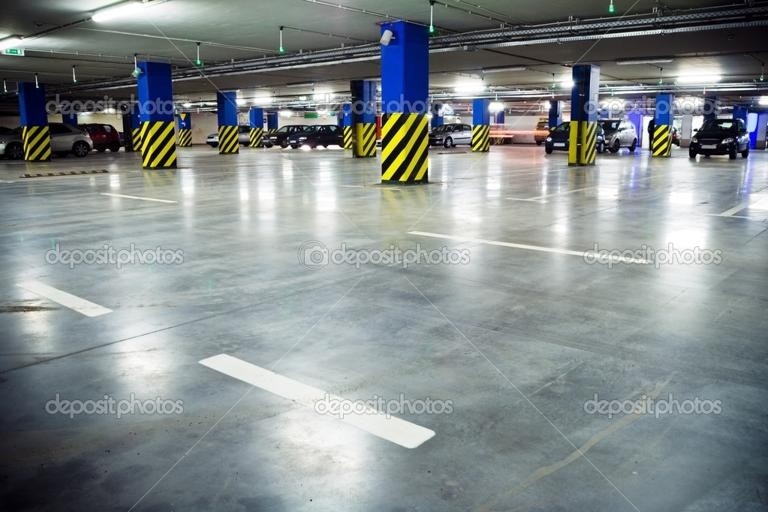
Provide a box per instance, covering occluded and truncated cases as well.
[205,126,344,149]
[0,123,125,160]
[429,123,473,149]
[688,119,751,159]
[544,118,639,154]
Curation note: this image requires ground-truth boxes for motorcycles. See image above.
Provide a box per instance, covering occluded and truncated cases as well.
[671,127,680,146]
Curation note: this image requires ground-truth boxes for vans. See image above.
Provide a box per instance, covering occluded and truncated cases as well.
[534,120,550,145]
